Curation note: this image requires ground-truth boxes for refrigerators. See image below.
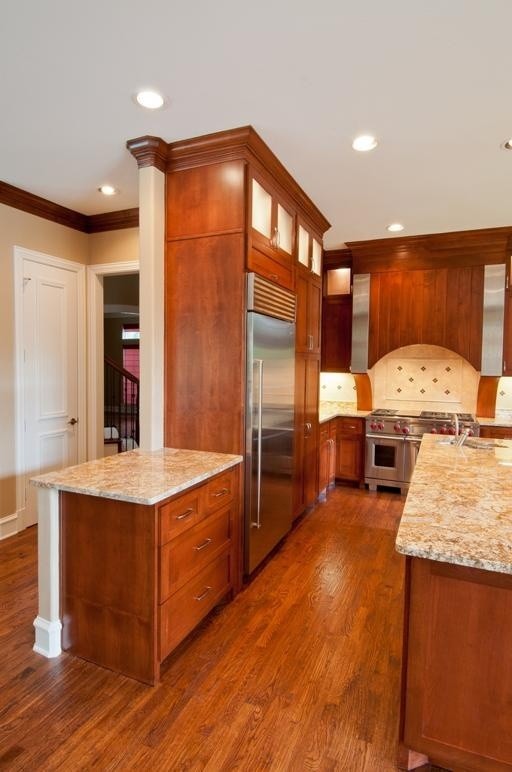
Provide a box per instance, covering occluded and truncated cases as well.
[244,311,297,577]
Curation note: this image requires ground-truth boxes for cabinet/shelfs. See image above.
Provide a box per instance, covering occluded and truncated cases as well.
[318,417,337,496]
[61,465,242,686]
[296,353,321,522]
[337,417,366,480]
[292,216,322,283]
[296,270,322,353]
[165,161,297,290]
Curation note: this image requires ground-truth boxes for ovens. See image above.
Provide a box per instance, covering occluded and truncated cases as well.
[363,420,474,495]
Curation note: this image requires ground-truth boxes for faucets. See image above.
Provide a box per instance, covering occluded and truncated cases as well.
[449,414,464,442]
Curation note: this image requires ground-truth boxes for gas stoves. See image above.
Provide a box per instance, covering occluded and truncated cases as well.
[372,408,474,421]
